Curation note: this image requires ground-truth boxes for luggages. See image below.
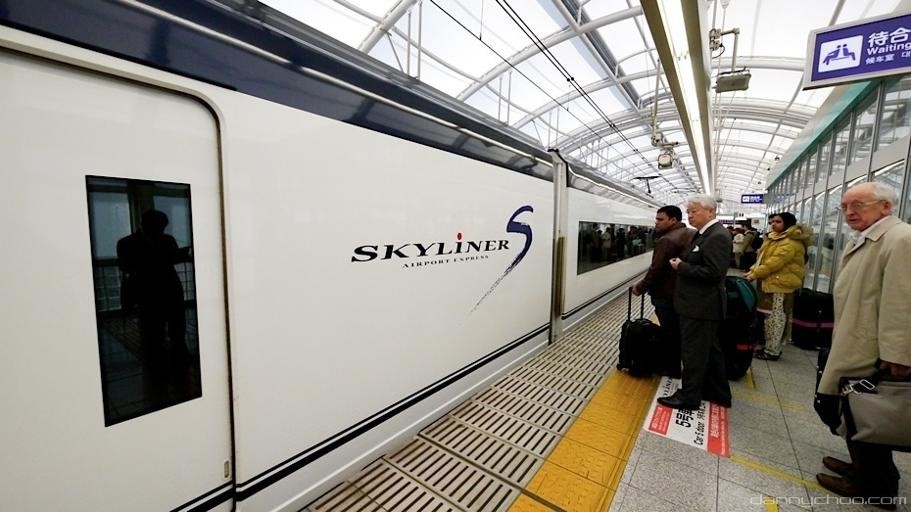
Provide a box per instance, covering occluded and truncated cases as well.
[616,284,659,378]
[791,288,833,350]
[720,286,758,381]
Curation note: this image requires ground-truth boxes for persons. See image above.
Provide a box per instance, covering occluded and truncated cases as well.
[657,194,734,411]
[725,219,764,272]
[581,222,656,262]
[816,180,911,510]
[742,212,794,344]
[633,206,697,378]
[116,210,195,409]
[743,212,813,360]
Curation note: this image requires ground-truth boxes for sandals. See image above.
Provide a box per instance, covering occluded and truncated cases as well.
[756,353,778,361]
[756,349,764,355]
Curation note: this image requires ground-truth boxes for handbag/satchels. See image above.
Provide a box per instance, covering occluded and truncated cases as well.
[725,275,757,316]
[837,367,910,453]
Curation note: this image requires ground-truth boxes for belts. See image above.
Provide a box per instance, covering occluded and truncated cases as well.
[813,350,843,434]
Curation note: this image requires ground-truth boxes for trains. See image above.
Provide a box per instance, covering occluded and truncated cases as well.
[0,1,690,510]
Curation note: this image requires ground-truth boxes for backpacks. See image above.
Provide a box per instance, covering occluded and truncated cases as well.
[746,232,763,250]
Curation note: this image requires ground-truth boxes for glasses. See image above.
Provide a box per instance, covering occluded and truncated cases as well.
[838,199,884,212]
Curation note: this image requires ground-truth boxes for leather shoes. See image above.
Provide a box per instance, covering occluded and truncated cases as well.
[815,473,896,510]
[657,391,702,410]
[822,456,866,479]
